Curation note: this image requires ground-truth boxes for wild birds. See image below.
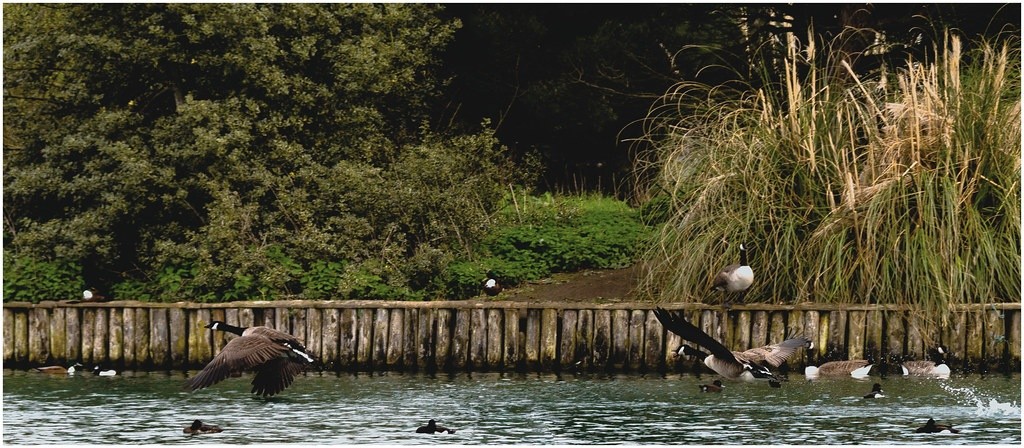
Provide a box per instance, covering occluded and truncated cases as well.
[916,417,960,434]
[805,359,877,379]
[709,242,754,309]
[652,305,810,385]
[416,419,455,435]
[769,374,785,388]
[65,286,113,304]
[182,321,316,398]
[91,365,121,377]
[482,269,502,302]
[901,345,952,376]
[36,361,83,374]
[864,382,885,399]
[183,420,223,435]
[698,379,726,394]
